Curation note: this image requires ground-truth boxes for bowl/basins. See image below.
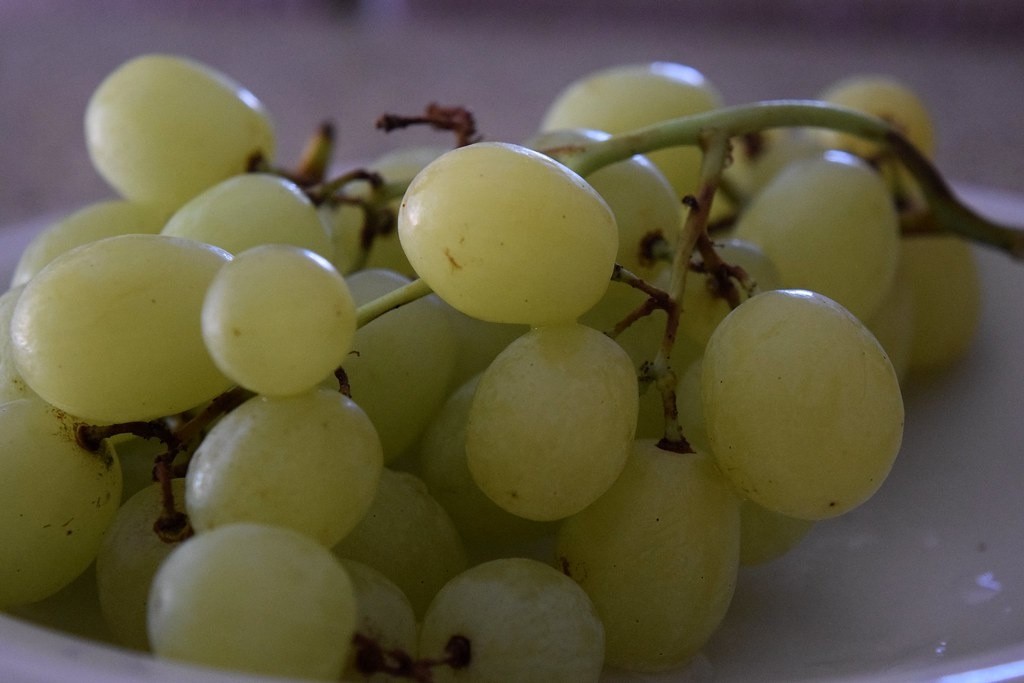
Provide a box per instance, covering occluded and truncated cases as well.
[0,163,1024,683]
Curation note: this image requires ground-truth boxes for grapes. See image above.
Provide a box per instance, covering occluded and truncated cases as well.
[0,53,1023,683]
